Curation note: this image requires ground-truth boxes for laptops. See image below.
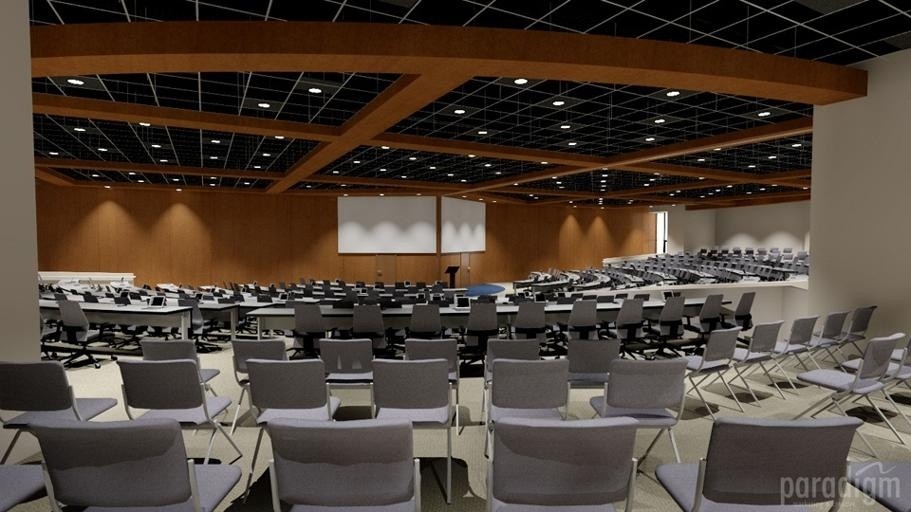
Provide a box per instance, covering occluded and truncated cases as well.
[143,296,165,309]
[274,294,288,302]
[662,290,674,303]
[455,297,470,311]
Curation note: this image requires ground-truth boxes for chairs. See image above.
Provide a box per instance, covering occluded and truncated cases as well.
[729,319,786,408]
[568,298,600,342]
[589,357,691,465]
[0,463,45,511]
[116,356,243,467]
[370,358,453,506]
[0,359,117,468]
[266,417,421,512]
[240,357,342,505]
[615,298,645,339]
[141,341,230,413]
[295,304,328,356]
[516,303,546,342]
[486,416,641,512]
[841,339,911,428]
[835,305,879,355]
[682,325,745,420]
[353,306,386,350]
[700,294,724,335]
[35,423,243,512]
[791,331,907,460]
[484,338,541,372]
[58,299,101,370]
[320,338,374,390]
[230,338,287,435]
[466,303,498,344]
[655,415,847,510]
[404,338,461,435]
[807,311,851,369]
[735,292,756,330]
[890,341,911,362]
[409,304,441,337]
[568,338,621,388]
[484,357,572,461]
[658,295,686,337]
[768,314,821,395]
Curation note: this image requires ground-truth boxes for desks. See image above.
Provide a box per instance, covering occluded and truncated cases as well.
[245,298,733,340]
[38,300,192,355]
